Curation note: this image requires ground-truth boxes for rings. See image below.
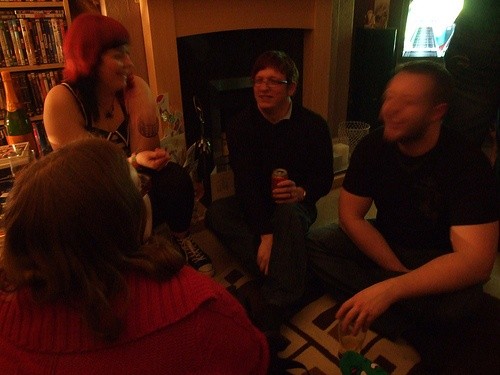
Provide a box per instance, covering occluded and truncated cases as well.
[289,192,292,199]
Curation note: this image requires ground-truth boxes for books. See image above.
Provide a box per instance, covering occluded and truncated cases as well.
[0,9,68,68]
[0,121,52,169]
[0,71,60,120]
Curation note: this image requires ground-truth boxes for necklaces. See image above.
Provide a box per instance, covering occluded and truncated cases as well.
[97,97,115,119]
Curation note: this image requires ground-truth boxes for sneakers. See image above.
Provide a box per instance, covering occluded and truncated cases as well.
[173,231,215,277]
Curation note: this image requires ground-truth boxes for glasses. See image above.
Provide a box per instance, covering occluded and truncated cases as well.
[252,77,288,88]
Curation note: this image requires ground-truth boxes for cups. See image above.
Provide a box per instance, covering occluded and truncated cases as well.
[338,320,365,358]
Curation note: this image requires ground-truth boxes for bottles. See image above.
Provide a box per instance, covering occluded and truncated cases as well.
[1,71,38,159]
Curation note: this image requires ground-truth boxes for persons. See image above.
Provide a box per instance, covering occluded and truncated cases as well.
[305,60,500,354]
[43,14,215,276]
[0,137,269,375]
[204,51,335,332]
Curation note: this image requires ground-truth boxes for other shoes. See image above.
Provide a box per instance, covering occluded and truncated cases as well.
[251,298,284,337]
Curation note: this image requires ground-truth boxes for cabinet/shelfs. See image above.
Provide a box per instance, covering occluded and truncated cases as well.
[0,0,72,159]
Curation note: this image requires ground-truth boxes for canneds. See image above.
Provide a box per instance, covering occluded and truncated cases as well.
[272,169,288,189]
